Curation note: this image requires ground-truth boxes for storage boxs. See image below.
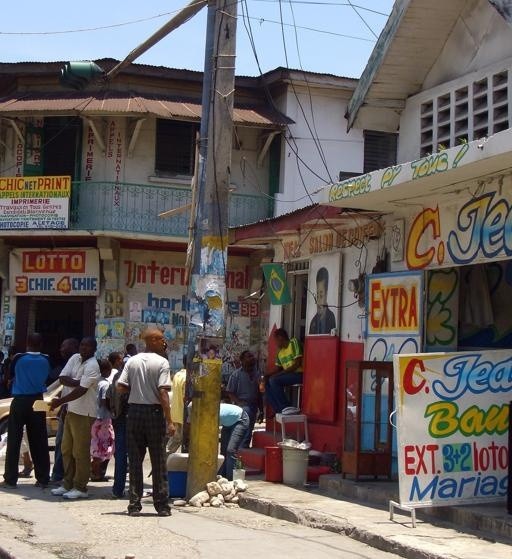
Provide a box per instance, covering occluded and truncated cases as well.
[166,453,225,498]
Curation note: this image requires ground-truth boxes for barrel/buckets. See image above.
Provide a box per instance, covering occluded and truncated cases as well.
[281,441,309,486]
[265,445,282,483]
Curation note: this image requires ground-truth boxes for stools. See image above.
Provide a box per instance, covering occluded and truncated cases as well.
[273,414,309,442]
[284,383,302,409]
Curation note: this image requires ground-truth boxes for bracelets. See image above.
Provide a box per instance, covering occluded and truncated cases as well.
[259,411,264,415]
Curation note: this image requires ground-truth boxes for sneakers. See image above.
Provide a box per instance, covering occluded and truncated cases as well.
[50,487,66,496]
[34,481,49,488]
[62,489,90,501]
[0,480,18,490]
[129,509,140,517]
[158,509,172,517]
[18,471,32,479]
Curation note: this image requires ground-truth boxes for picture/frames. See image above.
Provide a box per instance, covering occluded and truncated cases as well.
[304,251,343,337]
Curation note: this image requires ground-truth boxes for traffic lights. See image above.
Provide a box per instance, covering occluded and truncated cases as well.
[58,60,98,90]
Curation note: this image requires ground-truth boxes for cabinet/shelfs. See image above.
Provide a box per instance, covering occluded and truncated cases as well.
[340,359,394,484]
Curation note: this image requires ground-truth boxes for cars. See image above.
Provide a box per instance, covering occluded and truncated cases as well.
[0,374,64,440]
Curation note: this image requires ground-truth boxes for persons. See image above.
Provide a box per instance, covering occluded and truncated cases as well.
[308,266,336,334]
[166,354,188,459]
[259,327,304,416]
[185,400,251,481]
[0,327,173,517]
[221,350,264,455]
